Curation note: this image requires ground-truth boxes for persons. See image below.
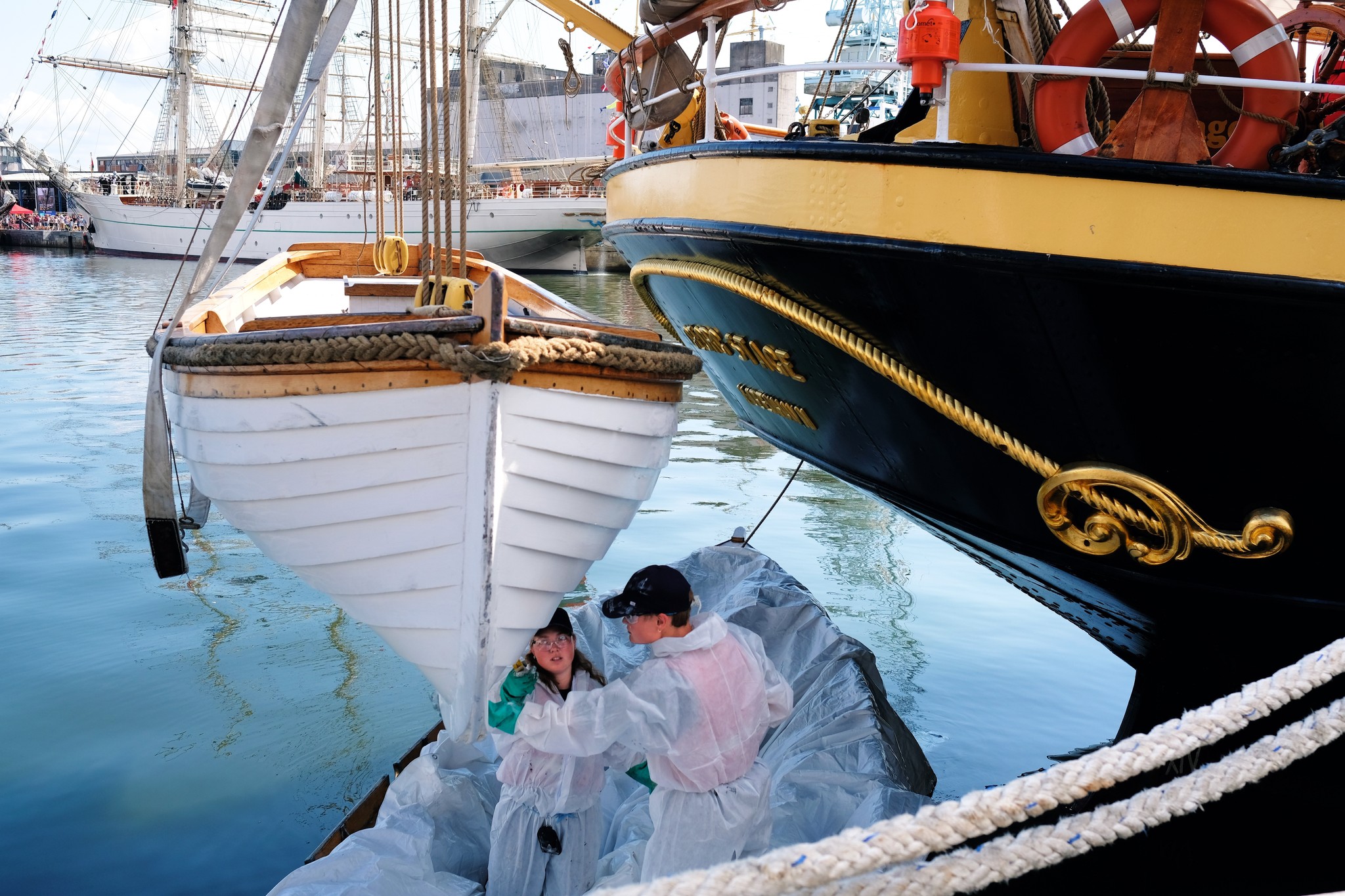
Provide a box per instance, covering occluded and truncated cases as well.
[487,565,794,883]
[97,175,137,196]
[396,177,414,201]
[486,606,657,896]
[0,214,87,232]
[38,196,53,205]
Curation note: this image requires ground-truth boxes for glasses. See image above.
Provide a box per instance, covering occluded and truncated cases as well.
[532,635,574,651]
[624,612,678,623]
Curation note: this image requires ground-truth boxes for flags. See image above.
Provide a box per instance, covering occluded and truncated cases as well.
[91,157,94,172]
[576,0,624,70]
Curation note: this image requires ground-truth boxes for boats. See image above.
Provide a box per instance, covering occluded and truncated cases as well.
[147,242,693,714]
[272,526,890,896]
[186,178,230,198]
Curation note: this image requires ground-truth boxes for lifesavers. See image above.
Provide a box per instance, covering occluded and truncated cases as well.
[502,186,512,198]
[1034,0,1301,171]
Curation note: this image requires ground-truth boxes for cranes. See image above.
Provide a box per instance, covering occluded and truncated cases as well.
[725,8,776,41]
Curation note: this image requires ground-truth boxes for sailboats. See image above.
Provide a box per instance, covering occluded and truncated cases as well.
[0,0,1345,896]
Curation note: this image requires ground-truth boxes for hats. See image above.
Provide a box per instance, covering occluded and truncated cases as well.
[535,607,573,633]
[601,565,694,619]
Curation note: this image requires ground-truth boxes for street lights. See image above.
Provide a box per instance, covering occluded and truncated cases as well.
[327,149,333,191]
[544,141,548,182]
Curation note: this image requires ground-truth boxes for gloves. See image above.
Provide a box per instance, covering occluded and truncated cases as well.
[501,661,540,697]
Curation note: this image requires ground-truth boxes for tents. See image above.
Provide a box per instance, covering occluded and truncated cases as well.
[7,203,35,229]
